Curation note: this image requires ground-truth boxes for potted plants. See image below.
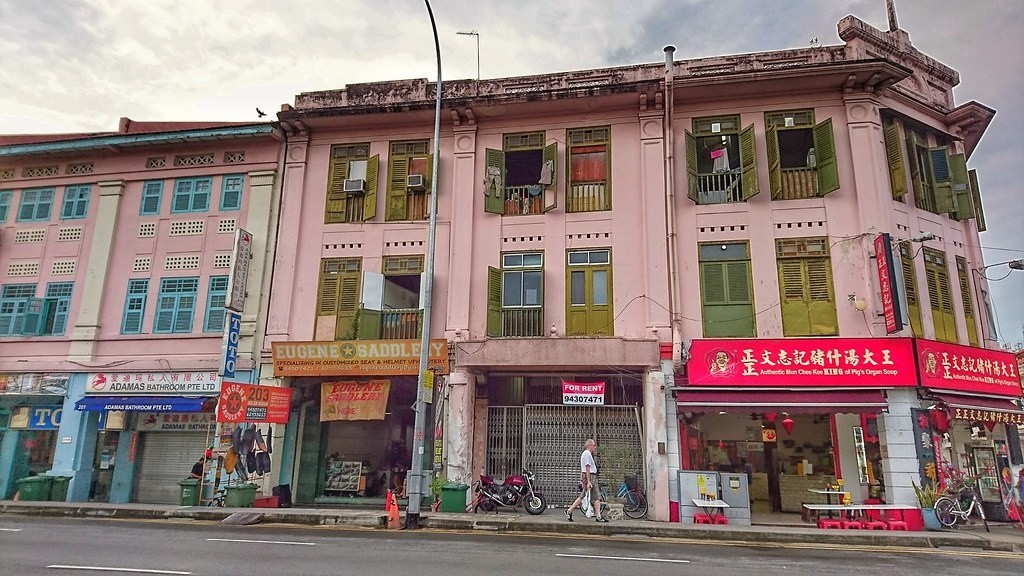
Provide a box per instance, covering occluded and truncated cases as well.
[597,443,642,521]
[911,476,942,530]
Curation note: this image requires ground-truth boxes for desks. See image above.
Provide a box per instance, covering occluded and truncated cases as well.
[692,498,731,523]
[803,504,919,521]
[807,489,847,519]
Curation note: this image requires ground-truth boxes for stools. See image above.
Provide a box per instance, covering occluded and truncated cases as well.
[817,517,908,531]
[693,513,728,525]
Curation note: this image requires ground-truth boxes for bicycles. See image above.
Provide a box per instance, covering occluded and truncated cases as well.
[579,473,648,519]
[434,480,496,516]
[933,476,990,532]
[581,475,639,512]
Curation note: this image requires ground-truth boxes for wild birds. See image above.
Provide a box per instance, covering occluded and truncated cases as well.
[257,107,268,119]
[810,37,819,45]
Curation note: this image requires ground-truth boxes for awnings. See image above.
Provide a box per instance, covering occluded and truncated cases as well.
[74,396,213,414]
[928,390,1024,424]
[675,389,891,418]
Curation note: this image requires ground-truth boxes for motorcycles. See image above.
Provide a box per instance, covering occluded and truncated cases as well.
[478,467,545,515]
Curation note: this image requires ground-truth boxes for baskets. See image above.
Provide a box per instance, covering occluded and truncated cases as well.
[625,475,638,491]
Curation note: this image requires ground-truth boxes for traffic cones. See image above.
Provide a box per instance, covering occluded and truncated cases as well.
[384,489,391,511]
[386,493,406,531]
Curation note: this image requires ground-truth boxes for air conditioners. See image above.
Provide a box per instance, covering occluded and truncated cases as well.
[406,174,425,192]
[344,178,366,194]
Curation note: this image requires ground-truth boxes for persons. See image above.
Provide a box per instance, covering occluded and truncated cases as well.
[563,439,609,523]
[191,457,206,478]
[741,458,752,485]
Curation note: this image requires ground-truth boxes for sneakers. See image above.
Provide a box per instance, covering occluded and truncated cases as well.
[563,510,574,522]
[596,515,610,523]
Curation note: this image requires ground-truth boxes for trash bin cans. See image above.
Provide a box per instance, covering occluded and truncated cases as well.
[15,475,54,502]
[176,478,211,508]
[439,482,470,514]
[48,475,75,503]
[223,482,260,507]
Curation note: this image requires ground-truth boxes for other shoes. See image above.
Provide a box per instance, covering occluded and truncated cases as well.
[749,497,755,503]
